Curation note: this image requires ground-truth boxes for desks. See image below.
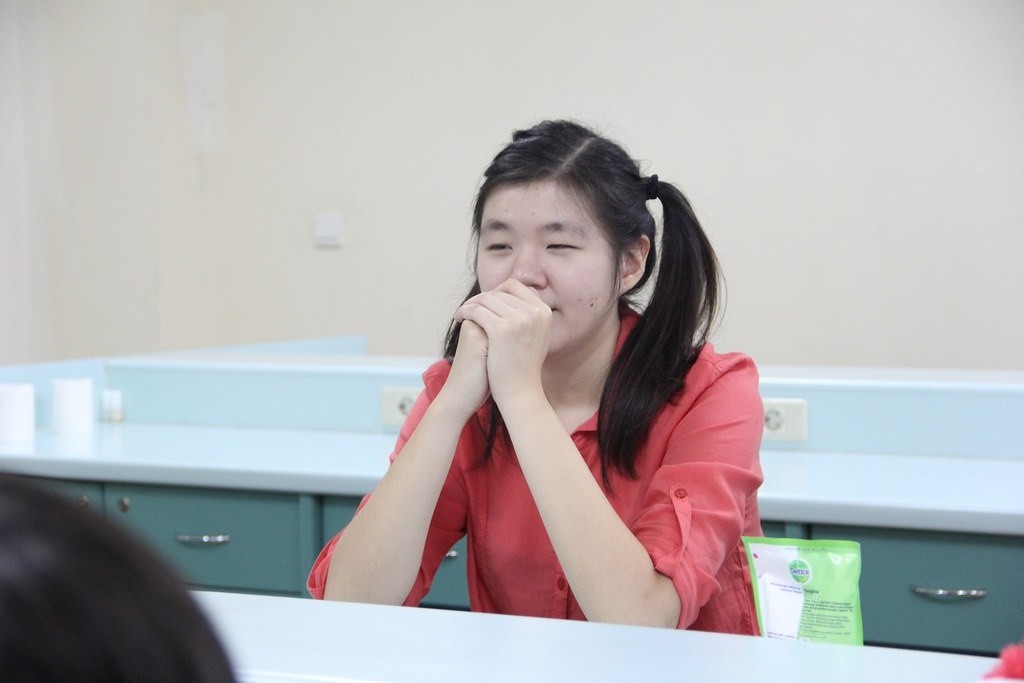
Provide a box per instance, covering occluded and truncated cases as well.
[1,425,1021,667]
[190,589,1005,680]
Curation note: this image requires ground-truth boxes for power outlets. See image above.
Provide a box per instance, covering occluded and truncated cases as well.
[381,385,425,424]
[760,398,809,442]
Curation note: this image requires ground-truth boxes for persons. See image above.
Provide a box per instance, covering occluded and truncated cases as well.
[0,474,238,683]
[307,119,769,638]
